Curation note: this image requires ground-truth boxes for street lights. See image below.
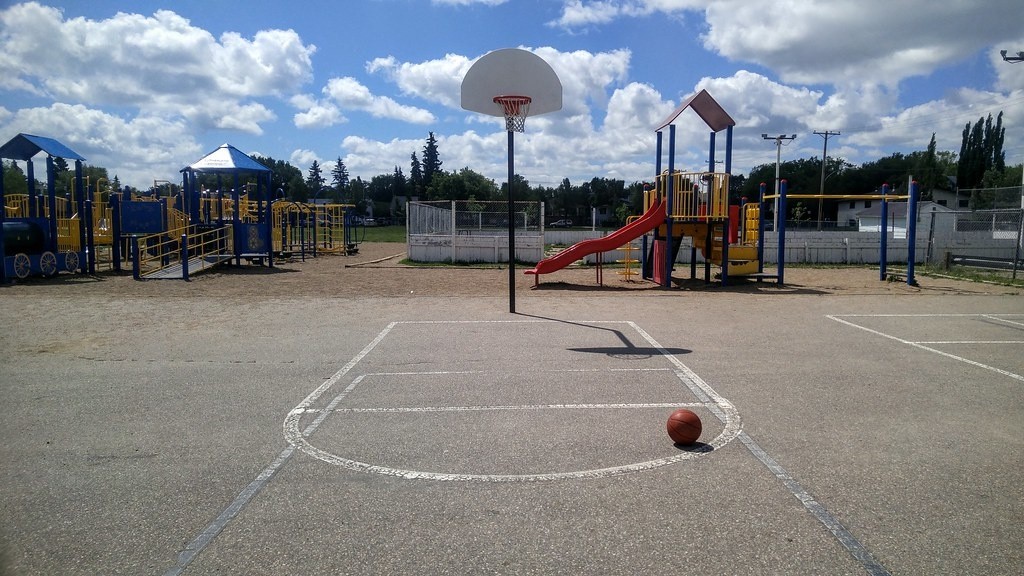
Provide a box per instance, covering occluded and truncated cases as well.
[762,134,796,231]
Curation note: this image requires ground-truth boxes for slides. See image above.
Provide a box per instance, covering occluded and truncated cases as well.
[526,198,665,277]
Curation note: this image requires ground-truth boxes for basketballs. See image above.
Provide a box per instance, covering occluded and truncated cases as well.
[667,409,703,445]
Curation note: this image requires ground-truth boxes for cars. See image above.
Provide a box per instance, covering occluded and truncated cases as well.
[304,217,330,227]
[364,219,374,228]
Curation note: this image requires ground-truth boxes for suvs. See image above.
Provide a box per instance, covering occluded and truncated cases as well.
[550,219,572,229]
